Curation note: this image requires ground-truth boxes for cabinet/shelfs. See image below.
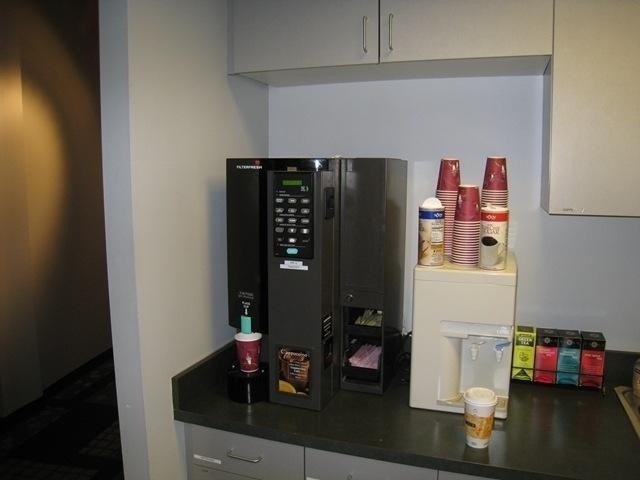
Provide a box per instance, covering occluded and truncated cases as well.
[182,423,494,479]
[226,0,553,87]
[541,0,640,218]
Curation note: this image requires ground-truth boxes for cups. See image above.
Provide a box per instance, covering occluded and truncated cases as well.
[479,236,505,268]
[464,387,497,450]
[278,348,310,390]
[234,332,262,373]
[435,155,509,264]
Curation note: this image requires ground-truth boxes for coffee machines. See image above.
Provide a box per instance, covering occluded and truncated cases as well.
[224,156,409,411]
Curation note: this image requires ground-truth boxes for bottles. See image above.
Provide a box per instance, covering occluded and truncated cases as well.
[417,205,446,268]
[479,207,511,272]
